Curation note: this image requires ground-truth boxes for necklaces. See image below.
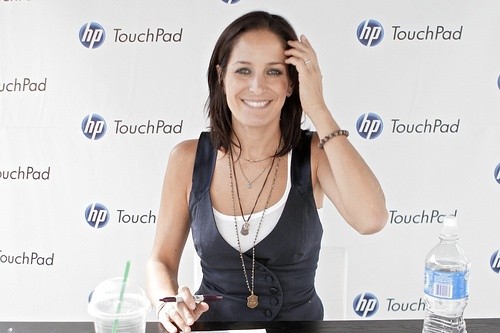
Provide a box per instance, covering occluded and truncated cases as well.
[225,134,280,309]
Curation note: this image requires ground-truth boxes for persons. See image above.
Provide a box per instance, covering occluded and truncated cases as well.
[147,11,389,333]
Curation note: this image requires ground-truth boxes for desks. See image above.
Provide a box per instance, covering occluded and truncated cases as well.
[0,318,500,333]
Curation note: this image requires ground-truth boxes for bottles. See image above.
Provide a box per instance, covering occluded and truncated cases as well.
[421,217,469,333]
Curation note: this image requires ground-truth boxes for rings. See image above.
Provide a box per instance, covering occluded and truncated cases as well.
[304,59,312,64]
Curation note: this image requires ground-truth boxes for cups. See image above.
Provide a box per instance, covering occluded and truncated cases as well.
[87,278,150,333]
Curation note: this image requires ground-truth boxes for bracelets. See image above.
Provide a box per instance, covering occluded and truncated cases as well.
[317,129,349,148]
[156,302,166,319]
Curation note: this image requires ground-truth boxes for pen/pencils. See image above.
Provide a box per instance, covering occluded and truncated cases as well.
[159,294,225,303]
